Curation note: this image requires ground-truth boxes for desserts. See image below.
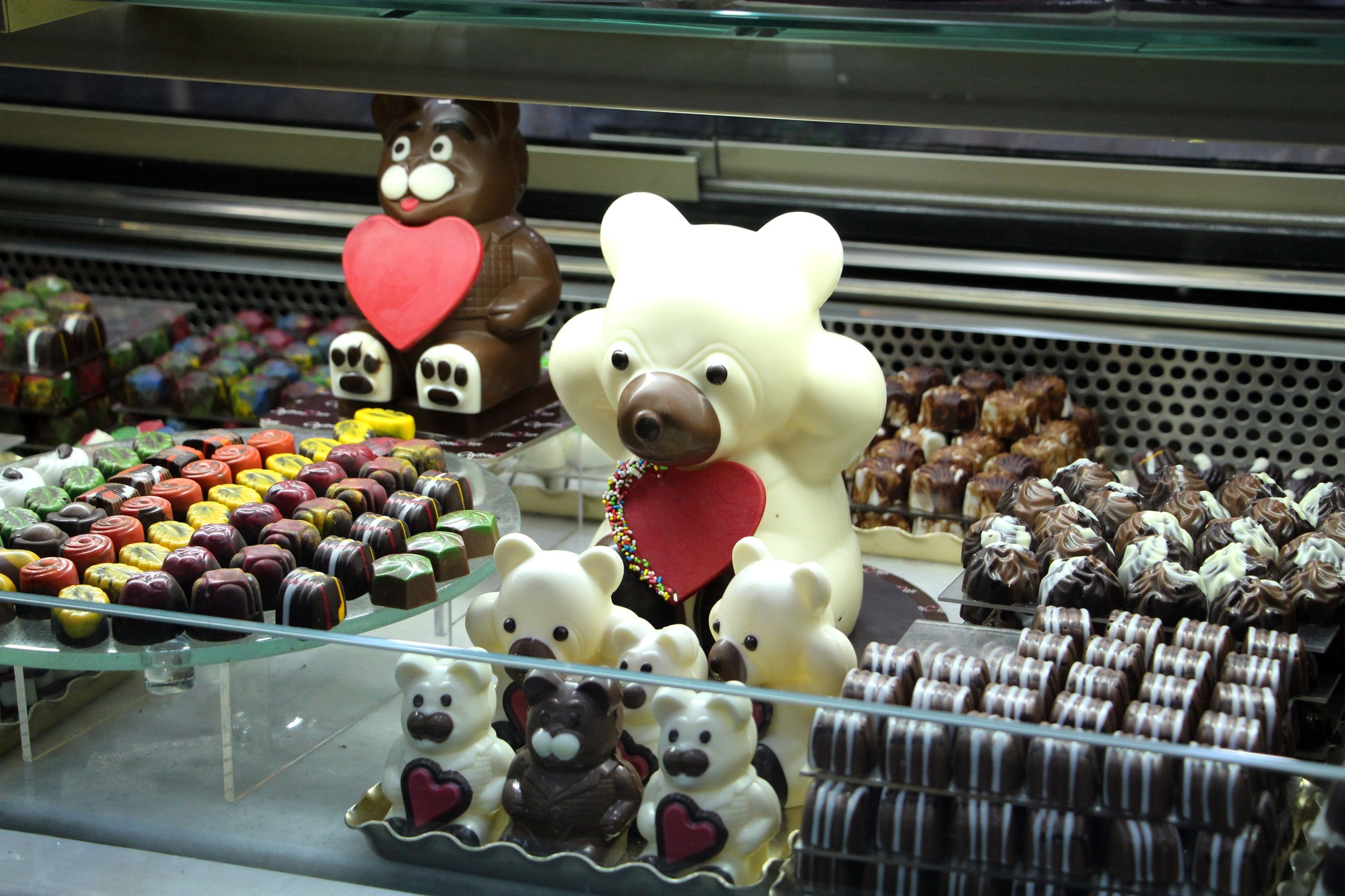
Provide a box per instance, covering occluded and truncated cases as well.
[0,664,86,723]
[787,597,1301,896]
[380,192,886,887]
[840,363,1345,633]
[0,431,501,647]
[328,94,561,420]
[0,274,361,419]
